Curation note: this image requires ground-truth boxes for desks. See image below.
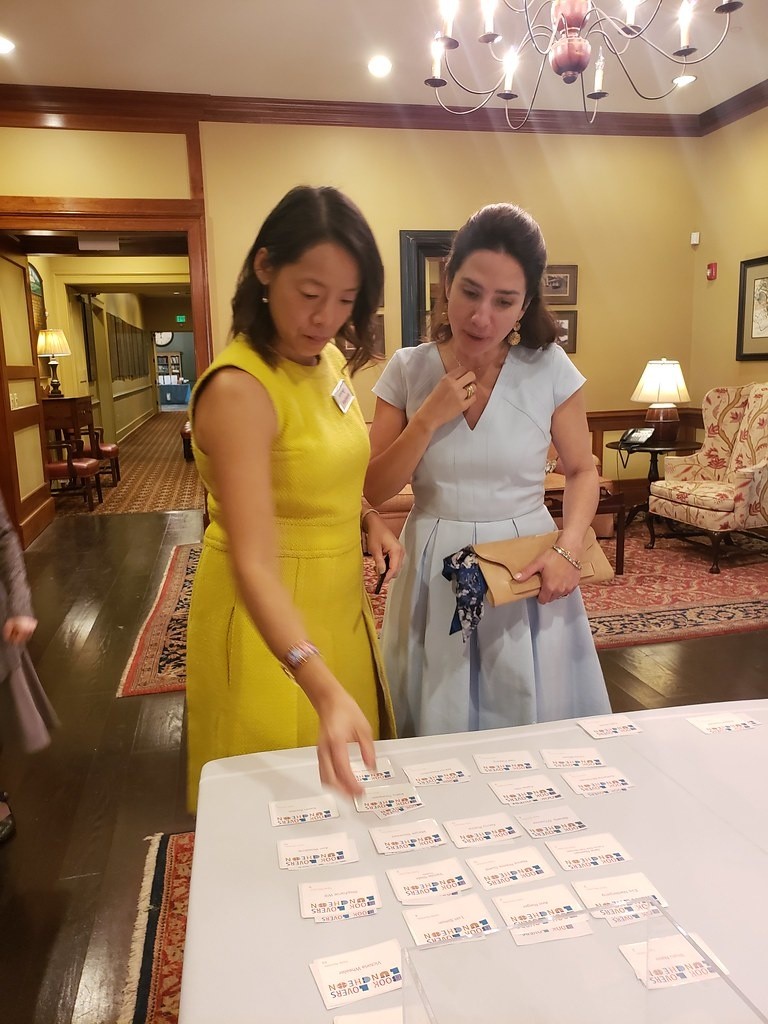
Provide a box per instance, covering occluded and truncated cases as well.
[177,699,768,1024]
[43,396,95,501]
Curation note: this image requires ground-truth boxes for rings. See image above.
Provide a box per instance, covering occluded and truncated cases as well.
[558,594,569,599]
[465,385,473,399]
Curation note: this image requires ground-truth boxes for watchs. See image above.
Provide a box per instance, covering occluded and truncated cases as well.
[280,640,321,682]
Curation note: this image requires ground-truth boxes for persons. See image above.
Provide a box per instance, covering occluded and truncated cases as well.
[183,187,405,814]
[0,494,62,845]
[363,201,599,739]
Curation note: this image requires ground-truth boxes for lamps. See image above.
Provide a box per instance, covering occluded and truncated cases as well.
[36,327,72,398]
[630,360,691,445]
[423,0,743,129]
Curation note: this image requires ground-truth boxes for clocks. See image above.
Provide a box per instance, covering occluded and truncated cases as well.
[155,332,173,346]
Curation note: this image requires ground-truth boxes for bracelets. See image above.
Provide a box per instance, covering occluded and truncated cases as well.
[549,543,582,570]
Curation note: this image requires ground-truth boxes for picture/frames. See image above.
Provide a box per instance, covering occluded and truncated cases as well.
[735,255,768,361]
[542,264,578,354]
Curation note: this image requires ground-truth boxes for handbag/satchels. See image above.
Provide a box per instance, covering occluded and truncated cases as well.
[469,526,615,608]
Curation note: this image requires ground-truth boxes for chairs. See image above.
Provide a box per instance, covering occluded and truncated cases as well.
[43,428,120,513]
[644,383,767,573]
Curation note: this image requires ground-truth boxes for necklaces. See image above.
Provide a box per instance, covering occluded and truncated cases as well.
[443,338,509,376]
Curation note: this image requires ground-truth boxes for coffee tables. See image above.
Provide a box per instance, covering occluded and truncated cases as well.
[606,442,703,533]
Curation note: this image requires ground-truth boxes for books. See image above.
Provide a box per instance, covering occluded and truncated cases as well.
[158,357,179,380]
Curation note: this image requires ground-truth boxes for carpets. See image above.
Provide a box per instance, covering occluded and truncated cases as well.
[115,511,767,696]
[115,832,194,1024]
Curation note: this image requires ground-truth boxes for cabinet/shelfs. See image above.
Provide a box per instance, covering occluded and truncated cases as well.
[156,352,183,377]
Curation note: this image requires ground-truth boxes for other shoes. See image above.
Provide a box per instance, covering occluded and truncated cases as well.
[0,790,15,842]
